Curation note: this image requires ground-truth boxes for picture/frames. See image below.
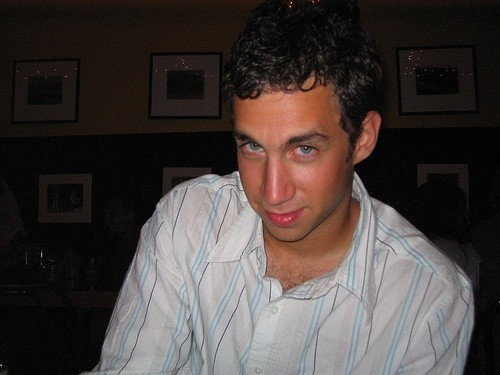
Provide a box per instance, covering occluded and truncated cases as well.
[148,51,224,120]
[394,44,481,117]
[10,57,81,125]
[160,164,213,200]
[414,161,474,227]
[34,172,95,226]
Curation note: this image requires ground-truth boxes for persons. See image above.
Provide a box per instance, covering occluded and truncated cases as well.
[80,0,474,375]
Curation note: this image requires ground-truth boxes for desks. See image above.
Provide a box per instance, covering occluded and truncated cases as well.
[0,291,117,375]
